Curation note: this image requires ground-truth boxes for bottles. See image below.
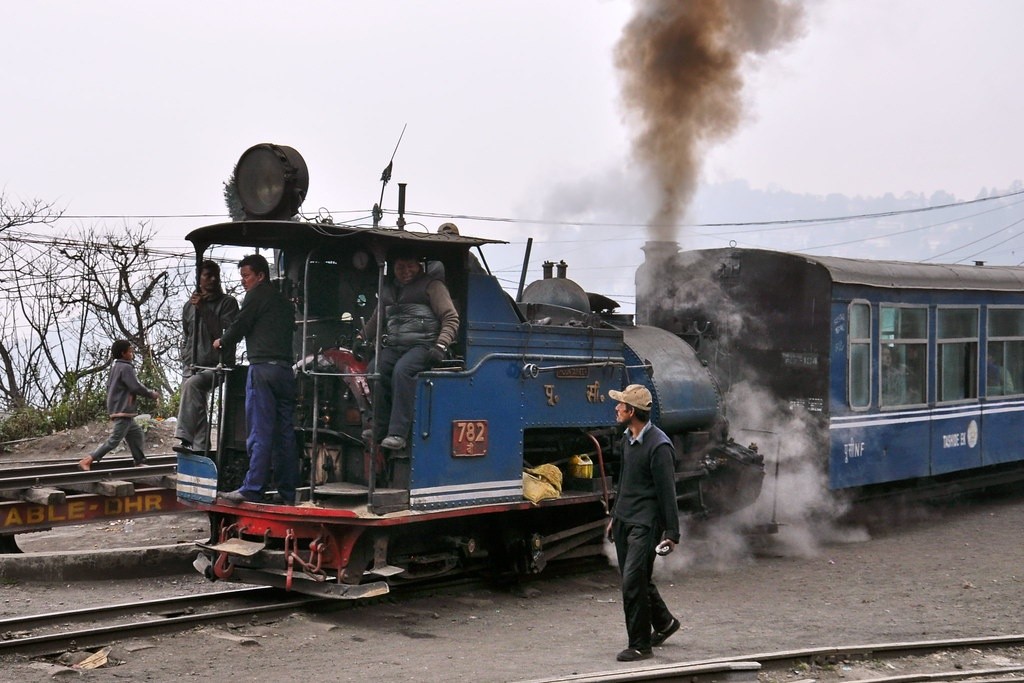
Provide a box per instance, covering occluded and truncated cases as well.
[569,454,594,479]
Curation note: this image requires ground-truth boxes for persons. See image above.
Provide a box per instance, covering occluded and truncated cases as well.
[213,254,297,506]
[79,340,159,471]
[881,344,1016,405]
[606,383,681,661]
[353,248,460,449]
[172,259,241,454]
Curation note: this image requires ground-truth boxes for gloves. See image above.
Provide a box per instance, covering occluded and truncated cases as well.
[351,338,364,361]
[428,343,444,365]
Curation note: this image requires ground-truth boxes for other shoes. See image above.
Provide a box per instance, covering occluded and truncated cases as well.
[646,620,681,647]
[361,428,372,438]
[382,435,406,448]
[174,441,194,453]
[261,492,295,506]
[618,646,654,661]
[215,489,252,501]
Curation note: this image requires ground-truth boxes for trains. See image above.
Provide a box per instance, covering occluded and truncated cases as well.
[175,123,1024,599]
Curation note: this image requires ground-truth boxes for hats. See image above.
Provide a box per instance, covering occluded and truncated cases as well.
[609,384,654,411]
[438,223,458,235]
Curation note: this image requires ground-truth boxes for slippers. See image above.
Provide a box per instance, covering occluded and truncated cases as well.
[77,464,84,471]
